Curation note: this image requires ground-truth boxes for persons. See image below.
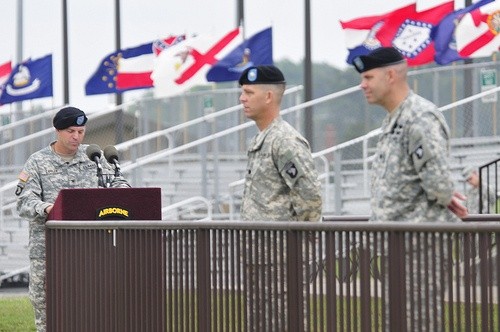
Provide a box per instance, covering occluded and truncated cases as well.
[460,167,494,251]
[352,49,468,332]
[237,65,322,332]
[18,107,131,332]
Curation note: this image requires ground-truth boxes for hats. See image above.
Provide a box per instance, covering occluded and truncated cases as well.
[352,46,406,73]
[238,65,286,86]
[53,107,87,130]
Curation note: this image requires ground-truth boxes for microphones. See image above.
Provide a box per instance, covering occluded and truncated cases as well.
[105,145,120,170]
[86,144,103,172]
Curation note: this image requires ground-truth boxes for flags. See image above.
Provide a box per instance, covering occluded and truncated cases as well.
[341,0,499,67]
[86,33,201,95]
[206,28,273,82]
[145,30,242,97]
[0,54,54,106]
